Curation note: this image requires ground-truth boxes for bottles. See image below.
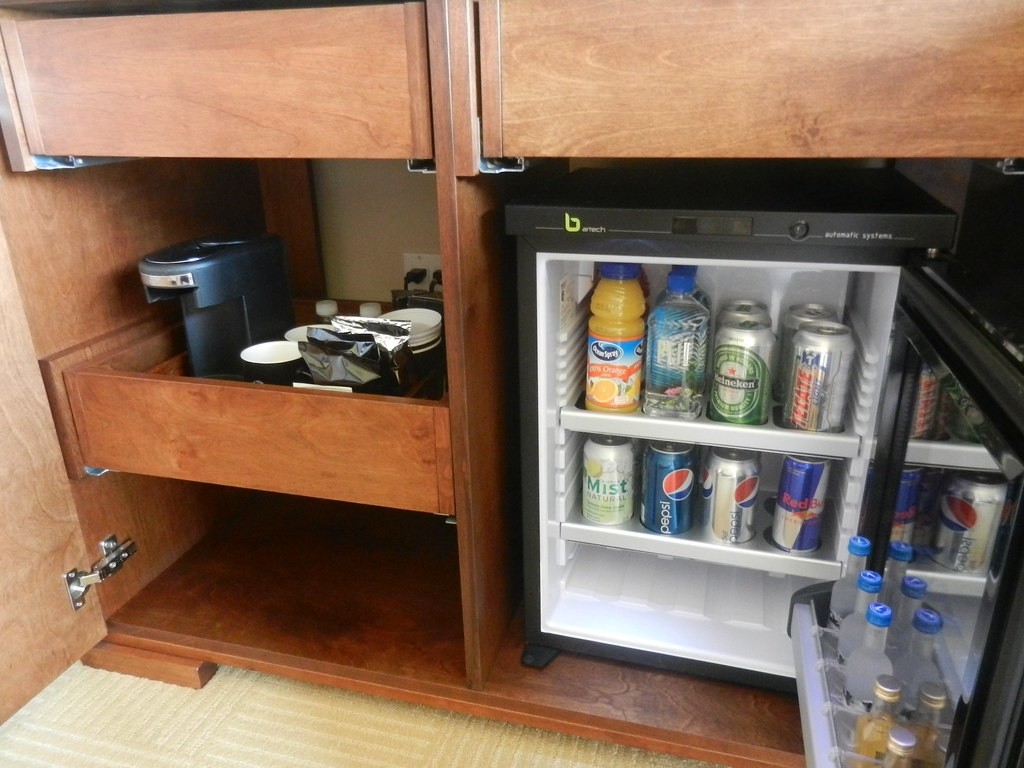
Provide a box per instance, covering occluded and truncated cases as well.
[851,673,902,768]
[842,602,893,750]
[584,263,712,419]
[906,680,947,768]
[835,570,882,705]
[884,577,928,662]
[825,536,871,663]
[315,300,341,323]
[882,727,917,768]
[892,607,938,723]
[878,540,912,622]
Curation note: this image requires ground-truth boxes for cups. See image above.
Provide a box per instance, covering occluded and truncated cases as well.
[285,324,334,342]
[240,341,312,387]
[378,307,442,377]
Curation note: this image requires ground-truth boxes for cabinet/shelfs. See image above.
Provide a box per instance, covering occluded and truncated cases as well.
[2,0,1024,768]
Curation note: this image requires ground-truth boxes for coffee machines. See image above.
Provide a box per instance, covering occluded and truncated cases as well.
[137,231,291,382]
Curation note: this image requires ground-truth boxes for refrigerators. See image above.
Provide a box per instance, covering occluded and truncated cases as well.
[502,159,1024,768]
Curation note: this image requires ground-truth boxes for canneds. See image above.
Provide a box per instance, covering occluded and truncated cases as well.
[889,360,1005,577]
[706,301,854,430]
[580,432,832,554]
